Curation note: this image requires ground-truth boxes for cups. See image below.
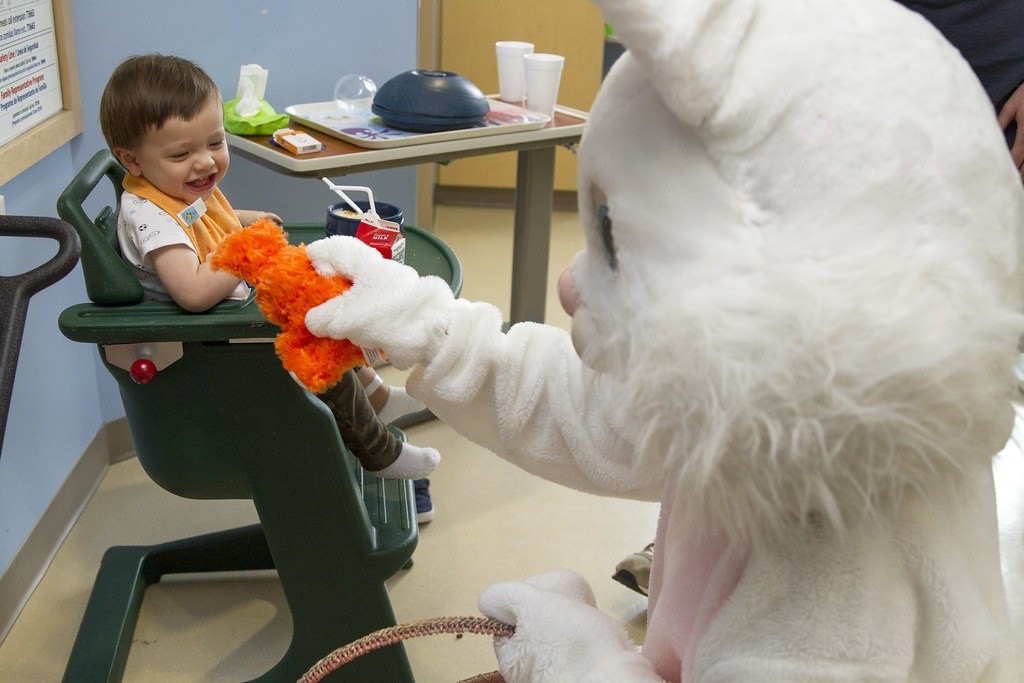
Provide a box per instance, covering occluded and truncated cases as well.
[495,41,534,102]
[524,54,565,123]
[325,200,404,258]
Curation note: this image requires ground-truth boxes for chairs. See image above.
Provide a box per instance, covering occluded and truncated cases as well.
[56,148,465,683]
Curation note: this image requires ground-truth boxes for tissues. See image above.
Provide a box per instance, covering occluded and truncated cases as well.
[222,62,291,135]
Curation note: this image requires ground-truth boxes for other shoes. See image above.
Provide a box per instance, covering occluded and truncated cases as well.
[413,477,434,522]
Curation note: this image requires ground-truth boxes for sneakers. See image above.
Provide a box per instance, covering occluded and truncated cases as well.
[612,541,653,591]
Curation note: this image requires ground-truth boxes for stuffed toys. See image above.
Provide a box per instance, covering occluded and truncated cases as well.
[311,0,1024,683]
[214,220,388,394]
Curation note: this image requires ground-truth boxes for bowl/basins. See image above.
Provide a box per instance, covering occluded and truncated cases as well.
[371,71,491,117]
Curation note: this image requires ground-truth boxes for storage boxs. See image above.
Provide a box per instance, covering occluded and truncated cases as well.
[272,127,321,155]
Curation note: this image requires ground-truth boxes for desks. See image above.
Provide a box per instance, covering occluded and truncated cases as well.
[222,94,589,427]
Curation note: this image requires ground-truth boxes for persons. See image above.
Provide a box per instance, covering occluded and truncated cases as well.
[99,54,440,482]
[611,0,1024,597]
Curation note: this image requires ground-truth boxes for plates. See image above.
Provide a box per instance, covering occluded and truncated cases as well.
[371,104,484,132]
